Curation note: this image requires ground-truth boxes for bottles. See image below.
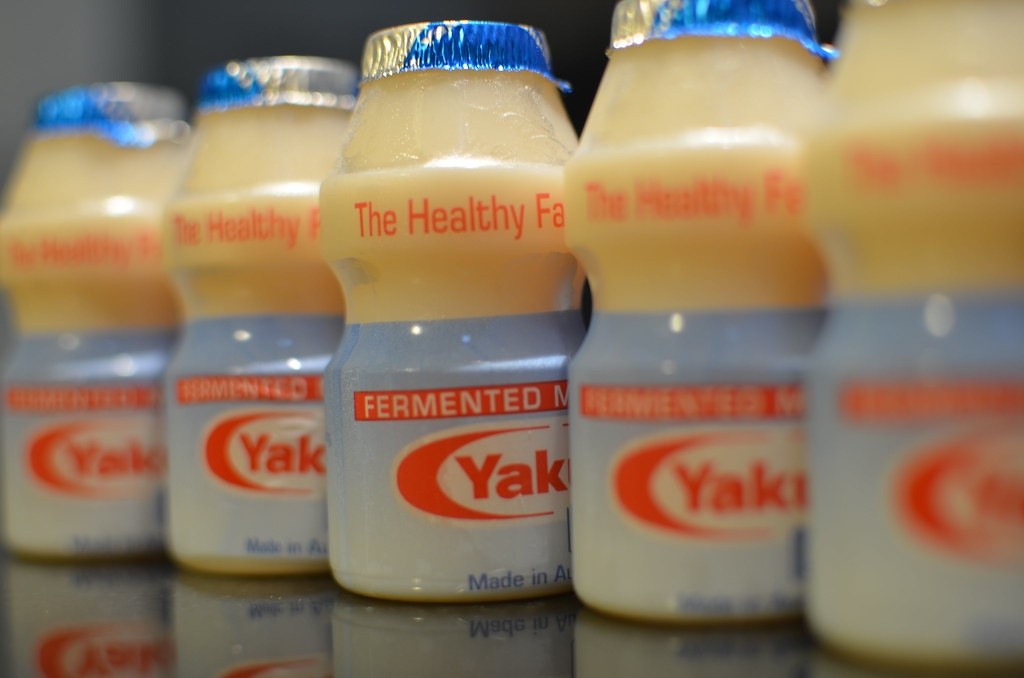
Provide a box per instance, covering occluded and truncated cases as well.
[564,0,828,625]
[0,83,183,564]
[809,0,1024,667]
[168,52,357,580]
[318,19,581,607]
